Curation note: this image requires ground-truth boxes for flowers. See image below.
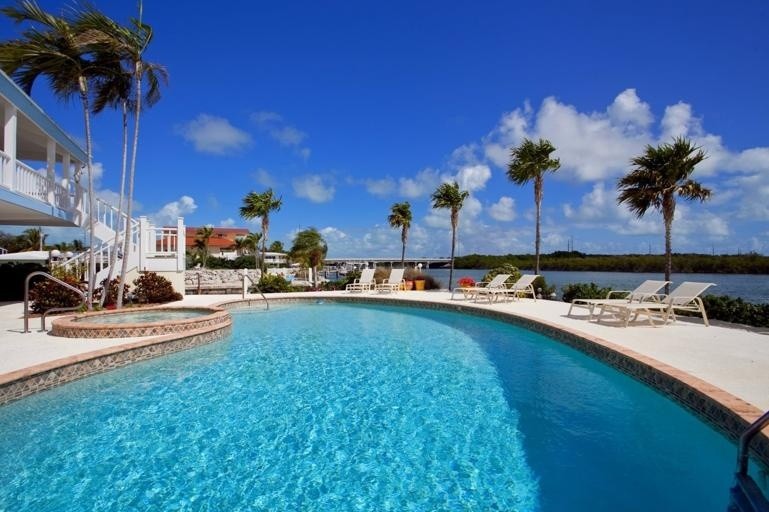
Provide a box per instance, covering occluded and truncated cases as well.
[458,277,474,286]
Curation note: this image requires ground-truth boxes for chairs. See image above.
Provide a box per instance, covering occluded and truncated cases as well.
[568,279,673,321]
[451,274,512,301]
[474,274,540,304]
[374,268,405,294]
[346,269,376,292]
[597,282,717,328]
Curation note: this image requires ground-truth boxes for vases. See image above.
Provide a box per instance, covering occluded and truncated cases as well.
[415,280,425,290]
[406,281,414,289]
[461,285,470,292]
